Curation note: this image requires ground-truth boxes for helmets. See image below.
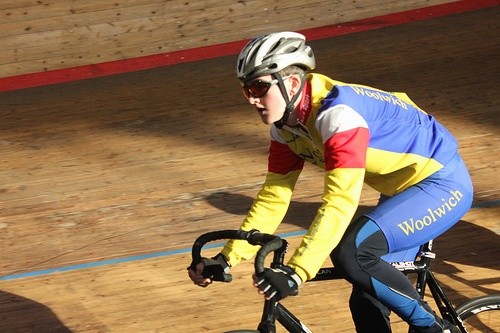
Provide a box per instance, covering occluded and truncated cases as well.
[237,31,317,82]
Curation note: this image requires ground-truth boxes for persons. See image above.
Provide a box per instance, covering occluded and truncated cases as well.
[187,30,474,333]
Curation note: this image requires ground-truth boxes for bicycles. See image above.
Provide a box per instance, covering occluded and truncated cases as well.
[190,227,500,333]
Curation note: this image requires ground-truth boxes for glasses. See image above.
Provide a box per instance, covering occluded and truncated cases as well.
[242,73,304,102]
